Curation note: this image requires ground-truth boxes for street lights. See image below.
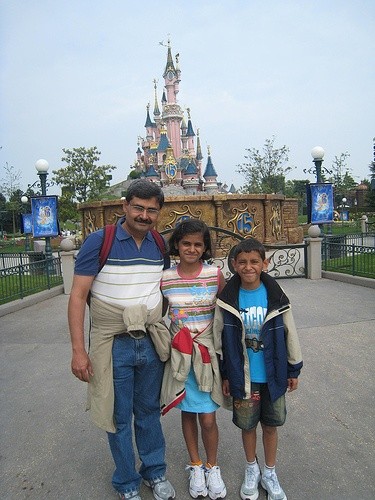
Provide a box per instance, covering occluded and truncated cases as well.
[21,195,31,252]
[311,146,325,255]
[35,159,57,275]
[342,197,346,210]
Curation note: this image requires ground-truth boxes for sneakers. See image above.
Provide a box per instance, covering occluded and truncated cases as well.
[142,473,175,500]
[185,463,208,499]
[260,469,287,500]
[204,463,227,500]
[240,464,262,500]
[118,489,141,500]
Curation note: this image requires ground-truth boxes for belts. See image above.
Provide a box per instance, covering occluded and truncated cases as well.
[117,329,144,337]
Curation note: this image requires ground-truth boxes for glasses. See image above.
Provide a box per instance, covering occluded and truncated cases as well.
[126,201,160,217]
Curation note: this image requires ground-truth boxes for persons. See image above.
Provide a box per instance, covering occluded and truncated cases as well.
[159,218,227,500]
[212,238,303,500]
[68,179,176,500]
